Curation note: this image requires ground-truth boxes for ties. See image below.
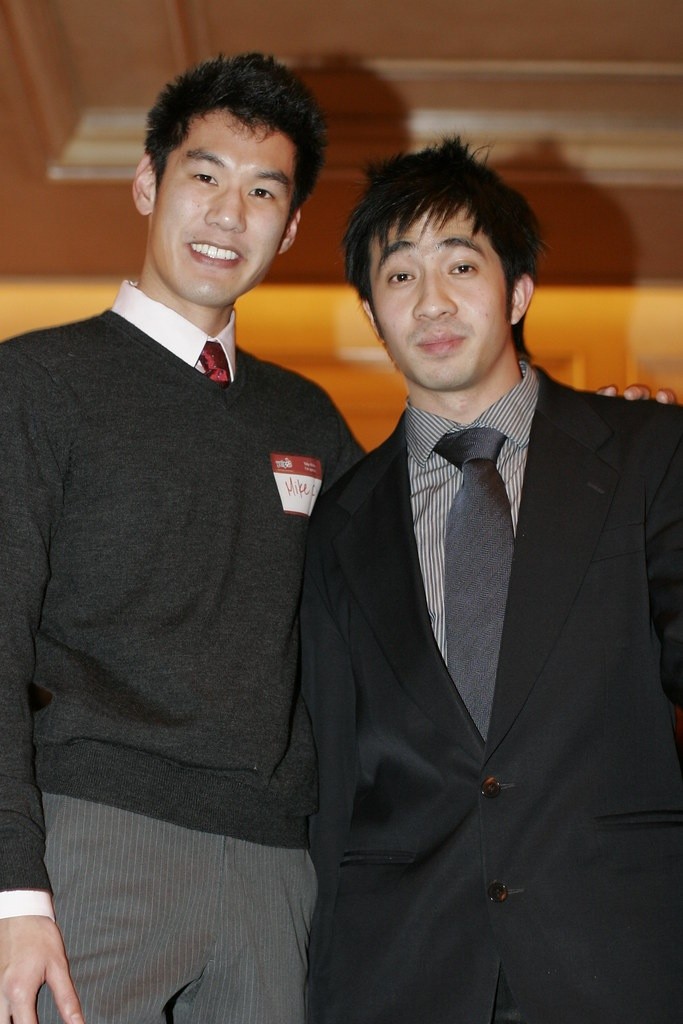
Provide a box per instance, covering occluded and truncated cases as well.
[199,341,232,391]
[431,426,517,740]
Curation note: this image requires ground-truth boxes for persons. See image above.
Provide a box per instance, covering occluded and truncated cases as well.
[0,48,677,1024]
[301,134,683,1024]
